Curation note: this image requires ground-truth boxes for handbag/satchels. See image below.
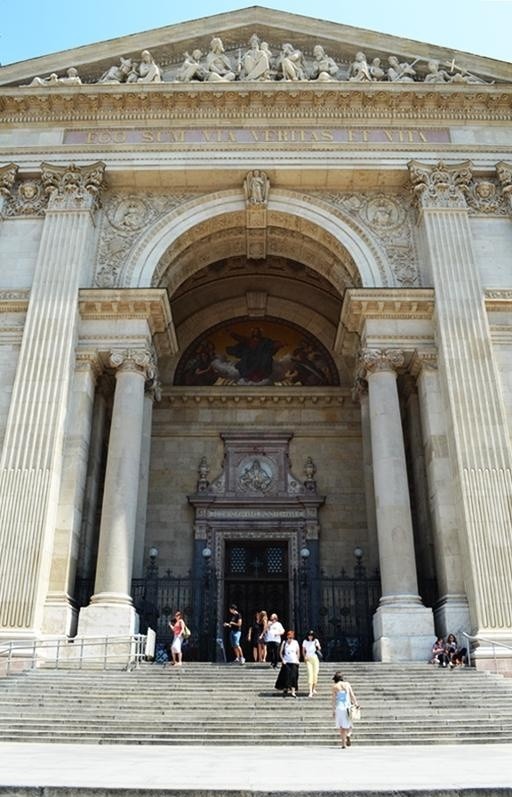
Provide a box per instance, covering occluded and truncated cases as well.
[180,621,191,640]
[347,705,360,720]
[278,647,284,656]
[316,649,323,658]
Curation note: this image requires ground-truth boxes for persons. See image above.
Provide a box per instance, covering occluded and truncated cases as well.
[100,49,162,83]
[257,610,268,662]
[180,31,340,82]
[167,608,186,666]
[275,631,301,697]
[263,613,286,669]
[346,51,419,83]
[444,634,467,667]
[302,629,322,698]
[177,323,330,387]
[223,603,246,665]
[331,672,360,749]
[239,460,273,490]
[424,59,497,84]
[18,67,83,87]
[248,611,260,661]
[432,638,457,668]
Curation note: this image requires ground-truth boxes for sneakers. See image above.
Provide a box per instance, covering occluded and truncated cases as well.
[283,689,317,698]
[342,735,350,747]
[235,656,245,664]
